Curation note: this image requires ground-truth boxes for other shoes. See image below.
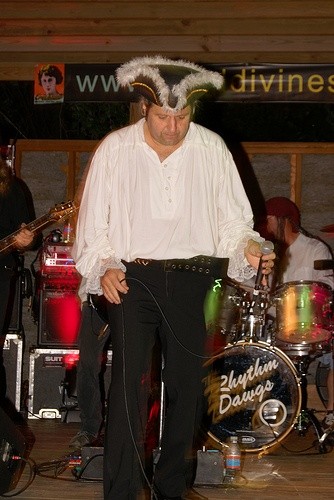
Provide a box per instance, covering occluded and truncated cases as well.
[72,430,97,446]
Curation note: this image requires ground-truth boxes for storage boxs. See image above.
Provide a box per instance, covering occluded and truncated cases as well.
[27,348,112,420]
[3,334,23,412]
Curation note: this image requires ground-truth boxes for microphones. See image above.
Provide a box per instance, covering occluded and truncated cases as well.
[253,239,274,289]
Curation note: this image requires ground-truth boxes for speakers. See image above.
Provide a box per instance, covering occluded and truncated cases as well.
[3,256,24,331]
[37,288,81,348]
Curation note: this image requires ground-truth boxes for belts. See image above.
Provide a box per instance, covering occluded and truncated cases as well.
[132,256,218,276]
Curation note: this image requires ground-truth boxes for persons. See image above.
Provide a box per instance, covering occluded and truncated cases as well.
[72,55,276,500]
[68,224,162,447]
[0,134,41,397]
[253,196,334,293]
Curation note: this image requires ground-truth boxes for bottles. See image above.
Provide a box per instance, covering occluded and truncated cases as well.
[63,217,75,243]
[227,436,242,485]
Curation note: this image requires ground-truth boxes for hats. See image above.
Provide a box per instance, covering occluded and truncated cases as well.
[116,55,224,111]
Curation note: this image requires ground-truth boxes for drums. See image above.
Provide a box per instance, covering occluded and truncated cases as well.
[269,322,330,356]
[275,279,332,346]
[199,340,304,452]
[202,277,272,344]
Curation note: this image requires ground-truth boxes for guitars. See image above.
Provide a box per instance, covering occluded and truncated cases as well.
[0,200,78,253]
